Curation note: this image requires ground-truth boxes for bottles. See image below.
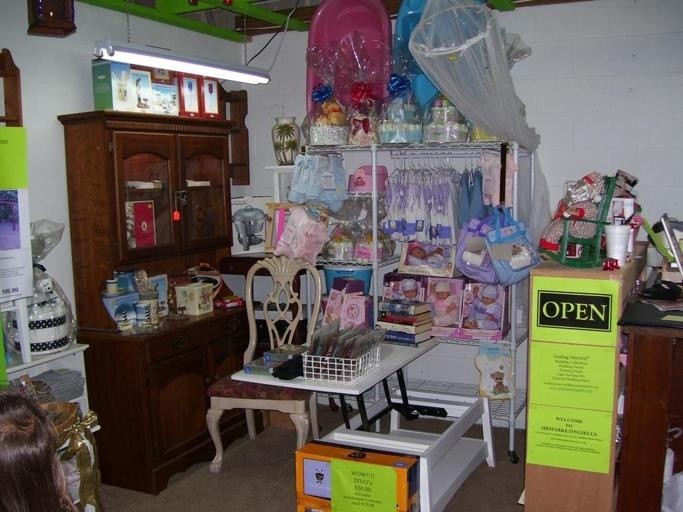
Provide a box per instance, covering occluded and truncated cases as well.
[271,117,300,165]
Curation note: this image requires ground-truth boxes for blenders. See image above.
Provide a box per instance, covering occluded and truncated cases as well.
[232,205,268,251]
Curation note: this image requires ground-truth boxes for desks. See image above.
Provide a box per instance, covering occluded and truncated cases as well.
[616,289,682,511]
[227,334,442,429]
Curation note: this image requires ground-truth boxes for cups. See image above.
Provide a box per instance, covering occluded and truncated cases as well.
[603,223,631,269]
[106,279,118,293]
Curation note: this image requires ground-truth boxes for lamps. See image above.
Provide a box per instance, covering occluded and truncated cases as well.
[91,1,272,86]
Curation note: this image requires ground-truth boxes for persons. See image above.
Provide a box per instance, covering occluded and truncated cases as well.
[400,278,420,300]
[409,247,444,264]
[431,283,459,326]
[466,286,502,329]
[0,394,78,512]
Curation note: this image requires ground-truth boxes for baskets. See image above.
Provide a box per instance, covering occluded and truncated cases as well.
[300,344,382,387]
[308,125,349,146]
[11,309,70,355]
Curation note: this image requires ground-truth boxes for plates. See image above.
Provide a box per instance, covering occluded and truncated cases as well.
[100,287,128,298]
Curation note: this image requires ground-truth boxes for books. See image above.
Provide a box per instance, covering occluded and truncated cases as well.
[375,298,437,348]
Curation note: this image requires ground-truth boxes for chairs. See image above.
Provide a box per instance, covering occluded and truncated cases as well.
[203,253,321,478]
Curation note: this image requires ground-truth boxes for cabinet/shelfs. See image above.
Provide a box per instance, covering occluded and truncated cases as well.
[54,108,272,496]
[260,136,534,466]
[5,339,102,493]
